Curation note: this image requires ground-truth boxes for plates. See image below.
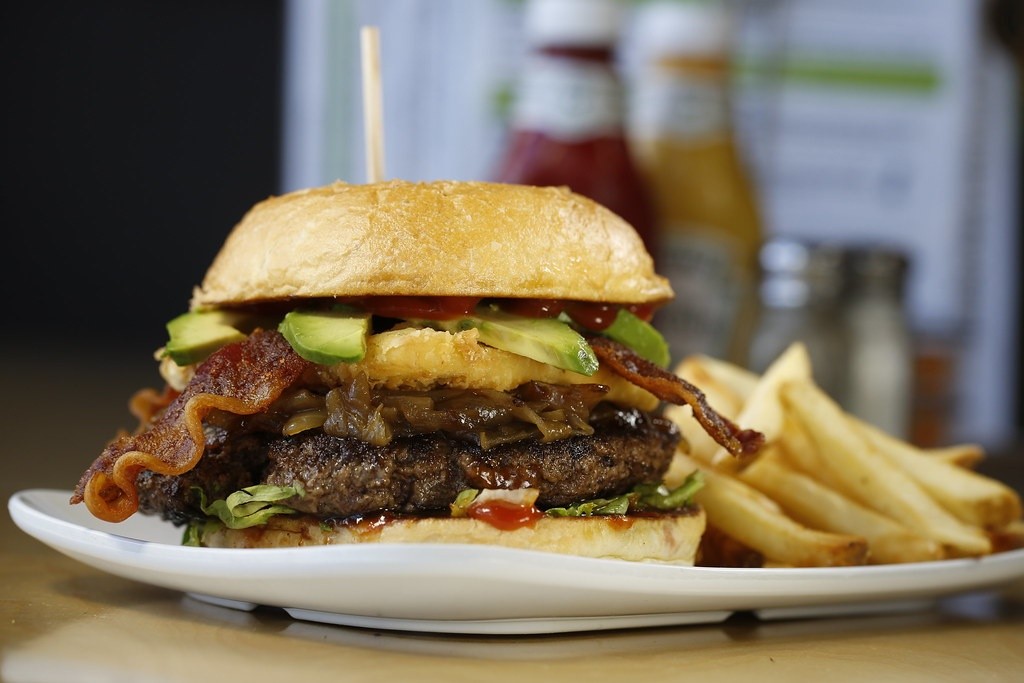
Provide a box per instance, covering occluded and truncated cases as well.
[9,488,1024,634]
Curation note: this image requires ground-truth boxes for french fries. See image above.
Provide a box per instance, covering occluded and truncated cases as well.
[664,341,1024,569]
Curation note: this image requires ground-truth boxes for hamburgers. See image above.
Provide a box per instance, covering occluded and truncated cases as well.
[65,181,763,568]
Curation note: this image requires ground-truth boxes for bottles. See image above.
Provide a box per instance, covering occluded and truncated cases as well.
[491,1,666,274]
[840,248,914,441]
[628,1,762,375]
[749,237,852,411]
